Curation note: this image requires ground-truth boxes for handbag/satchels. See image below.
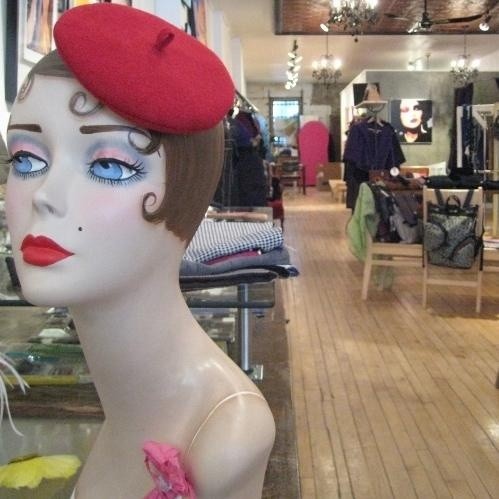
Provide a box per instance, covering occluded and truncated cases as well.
[372,185,482,269]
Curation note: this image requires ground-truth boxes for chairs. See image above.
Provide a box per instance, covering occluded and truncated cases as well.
[350,180,488,315]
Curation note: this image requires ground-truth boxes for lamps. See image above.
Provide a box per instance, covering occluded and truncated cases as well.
[447,21,482,90]
[475,13,492,32]
[319,0,382,45]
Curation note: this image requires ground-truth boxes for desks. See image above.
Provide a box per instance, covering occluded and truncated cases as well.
[0,196,286,420]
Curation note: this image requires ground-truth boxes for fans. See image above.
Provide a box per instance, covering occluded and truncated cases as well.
[383,0,487,37]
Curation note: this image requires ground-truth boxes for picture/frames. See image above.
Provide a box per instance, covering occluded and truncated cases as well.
[3,0,77,105]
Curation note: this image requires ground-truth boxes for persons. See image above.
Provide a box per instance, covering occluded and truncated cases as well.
[232,108,277,206]
[392,99,432,142]
[4,2,277,498]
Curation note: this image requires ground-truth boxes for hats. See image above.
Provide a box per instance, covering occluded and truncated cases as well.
[53,3,236,137]
